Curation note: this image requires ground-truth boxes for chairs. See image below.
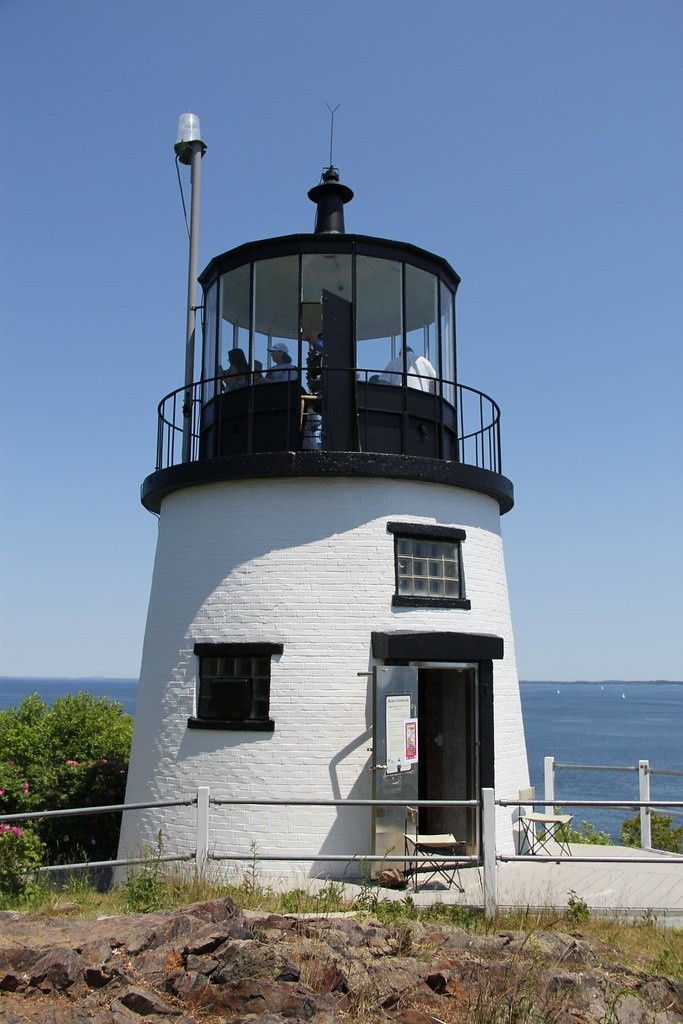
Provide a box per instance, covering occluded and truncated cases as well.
[405,806,466,894]
[516,785,573,864]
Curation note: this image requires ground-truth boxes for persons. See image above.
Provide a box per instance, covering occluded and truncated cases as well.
[379,345,436,394]
[254,343,298,383]
[218,349,249,392]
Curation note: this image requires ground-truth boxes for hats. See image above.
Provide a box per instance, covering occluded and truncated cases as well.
[267,343,290,355]
[247,360,262,371]
[399,345,413,356]
